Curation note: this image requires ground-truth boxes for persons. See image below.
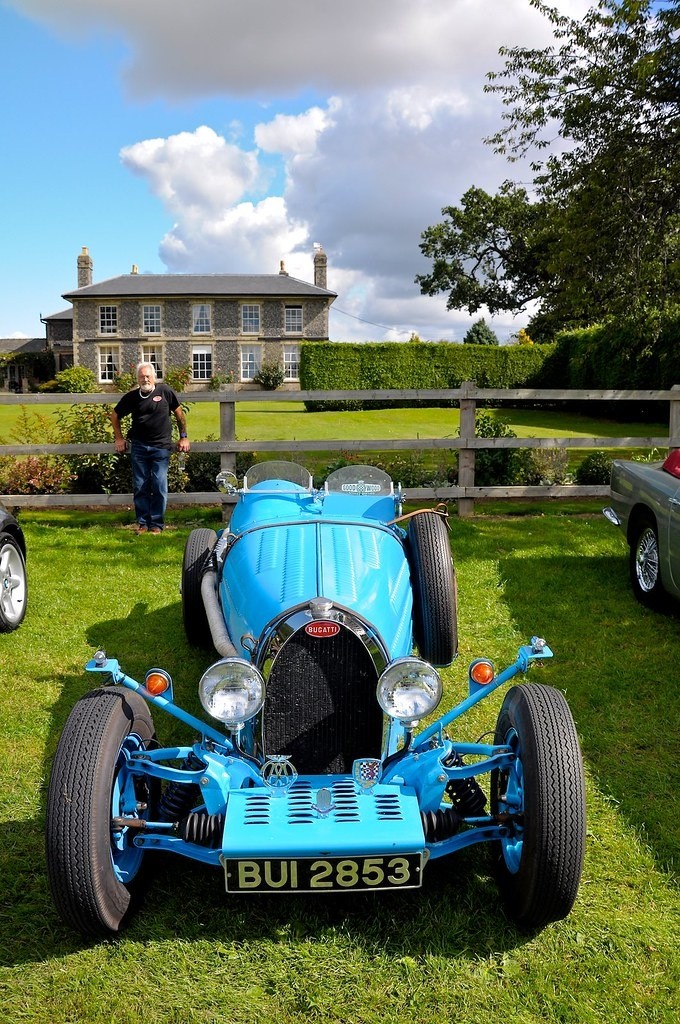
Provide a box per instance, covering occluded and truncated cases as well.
[111,362,191,536]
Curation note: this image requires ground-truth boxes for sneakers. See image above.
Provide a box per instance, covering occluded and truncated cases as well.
[136,527,149,535]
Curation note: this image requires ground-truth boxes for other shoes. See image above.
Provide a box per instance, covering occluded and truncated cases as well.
[150,527,161,535]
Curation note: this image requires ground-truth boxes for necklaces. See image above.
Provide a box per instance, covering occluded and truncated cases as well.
[139,387,155,398]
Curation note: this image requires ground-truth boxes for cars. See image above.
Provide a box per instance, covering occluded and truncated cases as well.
[48,460,587,948]
[0,503,35,632]
[604,444,680,608]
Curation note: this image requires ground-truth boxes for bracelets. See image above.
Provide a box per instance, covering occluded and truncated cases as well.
[181,433,187,438]
